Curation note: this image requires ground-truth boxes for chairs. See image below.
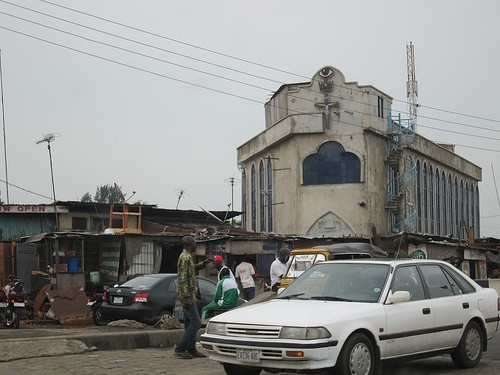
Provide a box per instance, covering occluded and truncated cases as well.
[329,274,364,296]
[427,274,452,296]
[397,268,416,291]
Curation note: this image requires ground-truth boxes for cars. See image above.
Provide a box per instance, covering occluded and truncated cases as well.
[102,273,250,328]
[196,259,500,375]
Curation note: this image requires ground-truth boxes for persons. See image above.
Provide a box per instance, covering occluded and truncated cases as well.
[171,236,210,359]
[235,254,255,301]
[271,247,290,291]
[208,255,235,282]
[201,268,240,321]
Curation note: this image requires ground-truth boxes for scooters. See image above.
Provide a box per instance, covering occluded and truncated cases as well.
[0,282,26,329]
[86,292,113,326]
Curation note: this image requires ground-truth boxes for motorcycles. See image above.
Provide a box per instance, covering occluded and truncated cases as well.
[271,243,390,303]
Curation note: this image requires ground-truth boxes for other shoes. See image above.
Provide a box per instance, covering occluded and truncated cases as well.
[191,348,206,357]
[175,347,192,359]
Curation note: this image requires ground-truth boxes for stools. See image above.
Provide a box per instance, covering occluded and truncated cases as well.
[107,204,142,235]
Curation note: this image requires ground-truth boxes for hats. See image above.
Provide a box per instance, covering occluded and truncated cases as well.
[208,255,223,262]
